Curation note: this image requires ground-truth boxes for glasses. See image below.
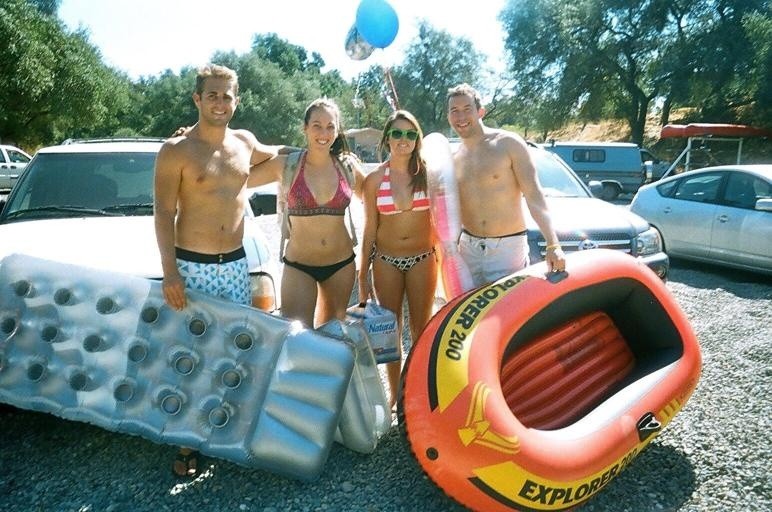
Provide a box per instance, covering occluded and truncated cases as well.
[387,128,419,141]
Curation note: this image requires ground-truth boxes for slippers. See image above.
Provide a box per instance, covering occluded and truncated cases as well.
[171,444,200,479]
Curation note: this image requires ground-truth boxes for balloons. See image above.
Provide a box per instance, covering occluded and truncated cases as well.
[343,26,376,62]
[356,0,399,49]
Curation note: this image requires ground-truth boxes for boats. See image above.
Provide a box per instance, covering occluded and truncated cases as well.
[395,246,704,511]
[1,253,359,486]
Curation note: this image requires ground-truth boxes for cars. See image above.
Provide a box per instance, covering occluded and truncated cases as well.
[0,139,279,318]
[631,162,772,273]
[1,146,34,192]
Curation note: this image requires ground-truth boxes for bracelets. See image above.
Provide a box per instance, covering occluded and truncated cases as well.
[545,244,560,252]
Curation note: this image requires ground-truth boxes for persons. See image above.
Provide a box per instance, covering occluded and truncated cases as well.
[170,96,366,333]
[151,61,361,482]
[444,82,566,288]
[358,110,444,413]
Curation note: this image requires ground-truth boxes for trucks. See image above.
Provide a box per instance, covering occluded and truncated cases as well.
[540,141,678,200]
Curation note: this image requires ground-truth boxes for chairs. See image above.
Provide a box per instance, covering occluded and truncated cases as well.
[62,175,119,210]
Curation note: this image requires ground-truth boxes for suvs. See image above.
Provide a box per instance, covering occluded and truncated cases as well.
[519,146,669,282]
